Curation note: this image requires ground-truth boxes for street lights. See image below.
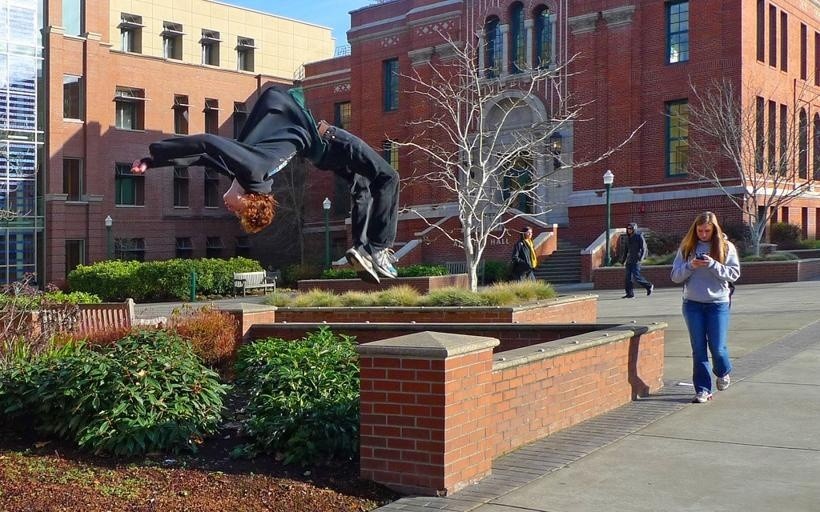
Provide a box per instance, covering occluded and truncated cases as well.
[322,198,331,271]
[603,170,614,267]
[105,215,113,260]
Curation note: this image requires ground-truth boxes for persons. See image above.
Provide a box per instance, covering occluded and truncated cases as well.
[670,211,741,403]
[130,86,400,284]
[620,223,654,298]
[513,227,538,281]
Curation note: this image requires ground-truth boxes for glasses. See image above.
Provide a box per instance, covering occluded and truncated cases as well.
[627,227,634,230]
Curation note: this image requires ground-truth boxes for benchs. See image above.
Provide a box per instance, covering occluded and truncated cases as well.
[232,269,276,299]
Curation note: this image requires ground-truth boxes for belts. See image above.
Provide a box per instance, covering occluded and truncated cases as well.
[318,119,329,137]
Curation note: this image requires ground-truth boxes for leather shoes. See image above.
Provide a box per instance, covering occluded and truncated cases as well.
[646,284,653,297]
[623,294,634,298]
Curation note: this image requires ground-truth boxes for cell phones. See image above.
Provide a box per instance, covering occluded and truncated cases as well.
[696,257,704,260]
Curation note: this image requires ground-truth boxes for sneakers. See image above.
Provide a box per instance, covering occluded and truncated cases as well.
[344,243,398,284]
[716,375,730,393]
[692,386,711,404]
